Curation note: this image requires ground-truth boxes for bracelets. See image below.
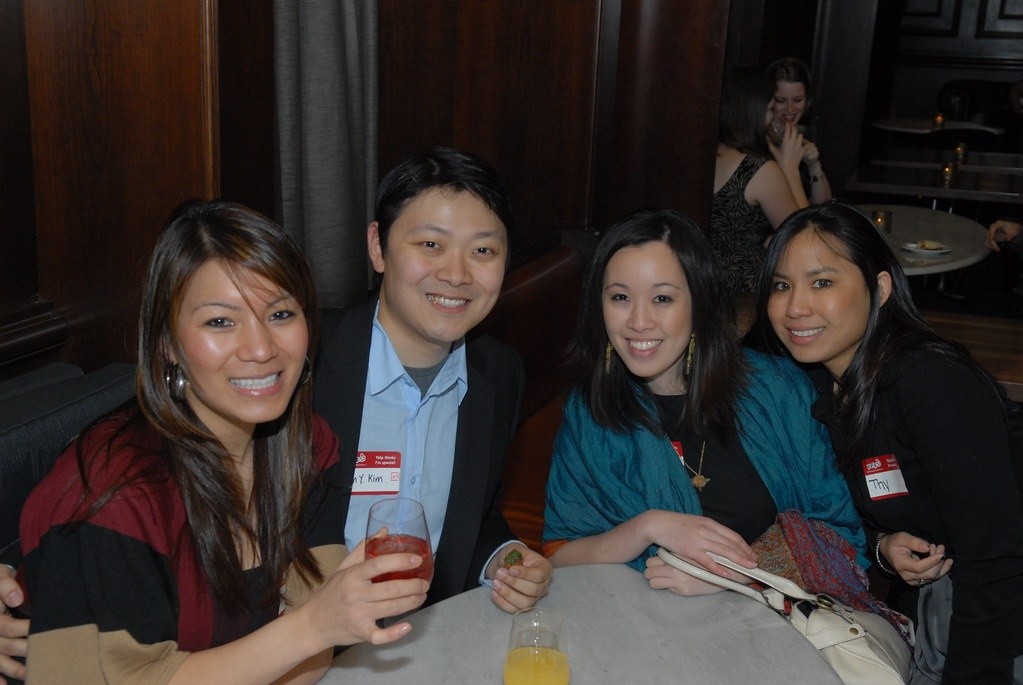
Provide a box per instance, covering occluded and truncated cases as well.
[875,532,899,576]
[808,172,825,185]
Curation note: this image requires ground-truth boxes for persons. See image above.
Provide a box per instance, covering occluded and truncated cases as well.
[710,55,833,322]
[983,219,1023,253]
[0,148,555,685]
[726,199,1023,685]
[5,201,430,685]
[528,207,869,600]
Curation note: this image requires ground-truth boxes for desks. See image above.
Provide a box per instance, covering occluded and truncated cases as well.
[846,204,992,276]
[871,118,1006,136]
[314,563,845,685]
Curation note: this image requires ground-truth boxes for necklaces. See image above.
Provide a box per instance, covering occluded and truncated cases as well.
[665,430,712,492]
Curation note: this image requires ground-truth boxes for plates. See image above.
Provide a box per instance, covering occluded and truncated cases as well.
[902,242,953,255]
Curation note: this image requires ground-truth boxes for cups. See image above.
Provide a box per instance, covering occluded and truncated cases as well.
[793,125,806,146]
[365,498,434,584]
[766,118,784,147]
[503,607,570,685]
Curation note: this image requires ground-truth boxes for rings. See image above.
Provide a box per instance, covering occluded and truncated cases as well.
[915,574,924,584]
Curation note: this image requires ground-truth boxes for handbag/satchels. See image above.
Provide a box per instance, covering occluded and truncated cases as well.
[655,543,912,685]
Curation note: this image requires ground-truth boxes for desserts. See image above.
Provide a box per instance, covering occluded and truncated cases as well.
[917,239,942,249]
